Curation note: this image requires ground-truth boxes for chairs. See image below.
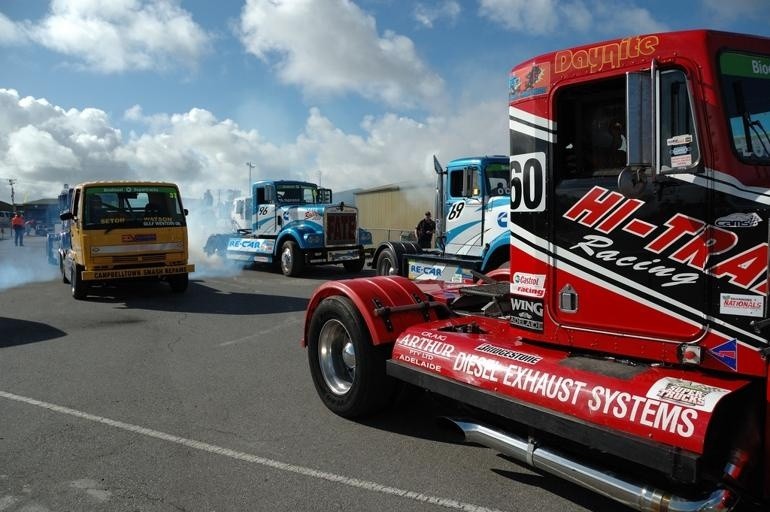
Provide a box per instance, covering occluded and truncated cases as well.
[88,195,111,223]
[592,132,626,169]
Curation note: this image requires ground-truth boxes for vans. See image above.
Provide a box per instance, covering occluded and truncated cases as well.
[0,211,24,228]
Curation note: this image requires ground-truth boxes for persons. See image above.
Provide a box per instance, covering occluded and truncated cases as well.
[12,212,24,247]
[25,220,34,234]
[416,211,436,248]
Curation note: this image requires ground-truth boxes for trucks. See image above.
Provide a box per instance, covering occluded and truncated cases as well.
[300,29,770,511]
[369,153,510,283]
[202,180,373,276]
[46,179,196,301]
[22,204,62,237]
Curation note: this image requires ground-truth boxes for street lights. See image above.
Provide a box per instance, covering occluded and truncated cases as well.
[246,161,256,192]
